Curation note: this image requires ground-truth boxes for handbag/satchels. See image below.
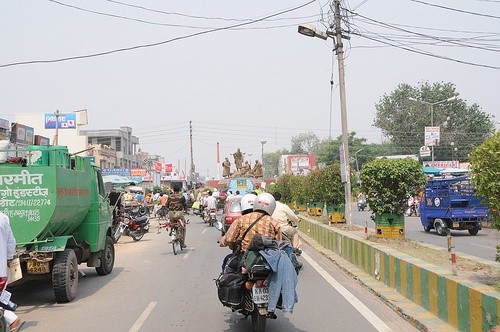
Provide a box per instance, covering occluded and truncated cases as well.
[221,253,241,273]
[239,251,302,279]
[192,201,200,208]
[214,274,249,309]
[248,234,288,251]
[233,241,241,255]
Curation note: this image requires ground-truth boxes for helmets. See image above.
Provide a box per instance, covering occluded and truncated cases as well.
[240,194,256,215]
[253,193,276,216]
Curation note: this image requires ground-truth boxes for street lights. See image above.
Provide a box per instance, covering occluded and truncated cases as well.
[261,140,267,159]
[355,143,372,189]
[408,95,458,161]
[54,109,62,146]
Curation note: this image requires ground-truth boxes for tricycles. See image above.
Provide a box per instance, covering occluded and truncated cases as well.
[419,177,490,237]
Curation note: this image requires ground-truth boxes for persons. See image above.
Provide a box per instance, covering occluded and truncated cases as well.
[223,157,230,177]
[0,212,25,332]
[124,191,168,215]
[357,192,366,206]
[218,189,300,312]
[233,148,242,170]
[182,187,227,221]
[243,160,262,175]
[165,185,187,248]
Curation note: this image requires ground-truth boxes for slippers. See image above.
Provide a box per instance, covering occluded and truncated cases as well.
[10,320,24,332]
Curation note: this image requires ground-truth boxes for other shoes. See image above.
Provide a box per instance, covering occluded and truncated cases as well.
[294,250,302,253]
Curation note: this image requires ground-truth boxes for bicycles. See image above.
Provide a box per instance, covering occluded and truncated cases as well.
[169,218,190,256]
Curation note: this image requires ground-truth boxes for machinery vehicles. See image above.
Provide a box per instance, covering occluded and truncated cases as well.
[0,148,116,303]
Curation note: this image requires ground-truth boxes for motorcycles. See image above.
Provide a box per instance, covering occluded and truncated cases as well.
[0,255,19,332]
[113,215,150,244]
[213,220,271,332]
[208,207,218,226]
[357,196,366,211]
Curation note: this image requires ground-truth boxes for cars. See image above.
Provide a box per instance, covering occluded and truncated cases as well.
[221,198,244,236]
